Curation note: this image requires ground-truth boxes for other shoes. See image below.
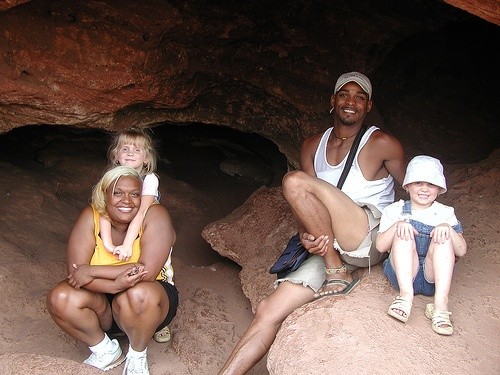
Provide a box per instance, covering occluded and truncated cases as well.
[153,325,171,343]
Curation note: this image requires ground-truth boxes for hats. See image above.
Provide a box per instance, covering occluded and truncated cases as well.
[402,154,448,195]
[333,71,373,100]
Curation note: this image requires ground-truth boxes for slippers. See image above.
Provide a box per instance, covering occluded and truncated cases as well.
[387,293,415,324]
[425,302,453,336]
[312,274,361,299]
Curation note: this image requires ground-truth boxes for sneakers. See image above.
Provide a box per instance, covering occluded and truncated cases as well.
[122,347,150,375]
[82,338,127,372]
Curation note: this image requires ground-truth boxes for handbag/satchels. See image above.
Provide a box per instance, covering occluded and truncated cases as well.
[269,232,311,279]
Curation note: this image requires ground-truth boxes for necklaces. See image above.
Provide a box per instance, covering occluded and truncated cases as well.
[332,129,358,140]
[139,166,144,174]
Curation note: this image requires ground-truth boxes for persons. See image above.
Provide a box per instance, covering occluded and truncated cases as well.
[375,155,467,336]
[99,125,170,343]
[45,167,180,375]
[215,72,410,375]
